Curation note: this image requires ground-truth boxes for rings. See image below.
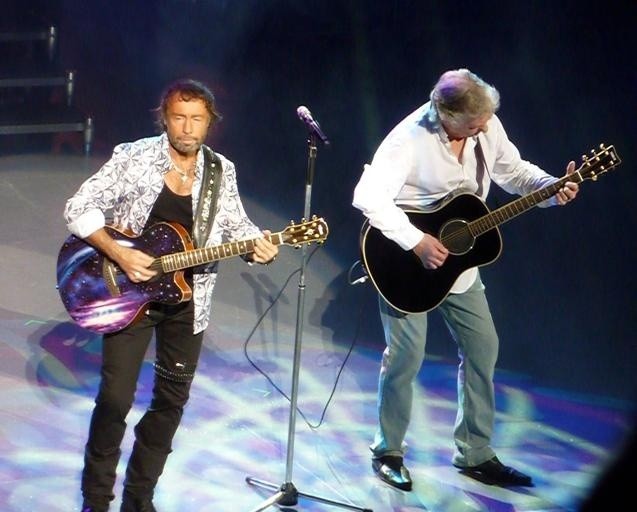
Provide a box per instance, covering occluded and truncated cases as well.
[132,272,142,279]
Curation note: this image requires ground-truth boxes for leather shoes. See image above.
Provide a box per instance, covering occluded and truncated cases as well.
[451,456,533,488]
[372,457,415,490]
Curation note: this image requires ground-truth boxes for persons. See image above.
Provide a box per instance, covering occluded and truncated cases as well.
[351,66,580,491]
[63,77,282,512]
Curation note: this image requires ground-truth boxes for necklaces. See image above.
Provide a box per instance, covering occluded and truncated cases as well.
[171,161,195,184]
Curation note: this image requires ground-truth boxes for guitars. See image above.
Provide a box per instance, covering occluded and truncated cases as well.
[56,215,329,335]
[357,143,621,314]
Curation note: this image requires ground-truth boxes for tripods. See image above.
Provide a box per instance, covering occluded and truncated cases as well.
[246,146,373,512]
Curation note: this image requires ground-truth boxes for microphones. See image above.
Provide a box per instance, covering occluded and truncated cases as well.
[296,105,330,145]
